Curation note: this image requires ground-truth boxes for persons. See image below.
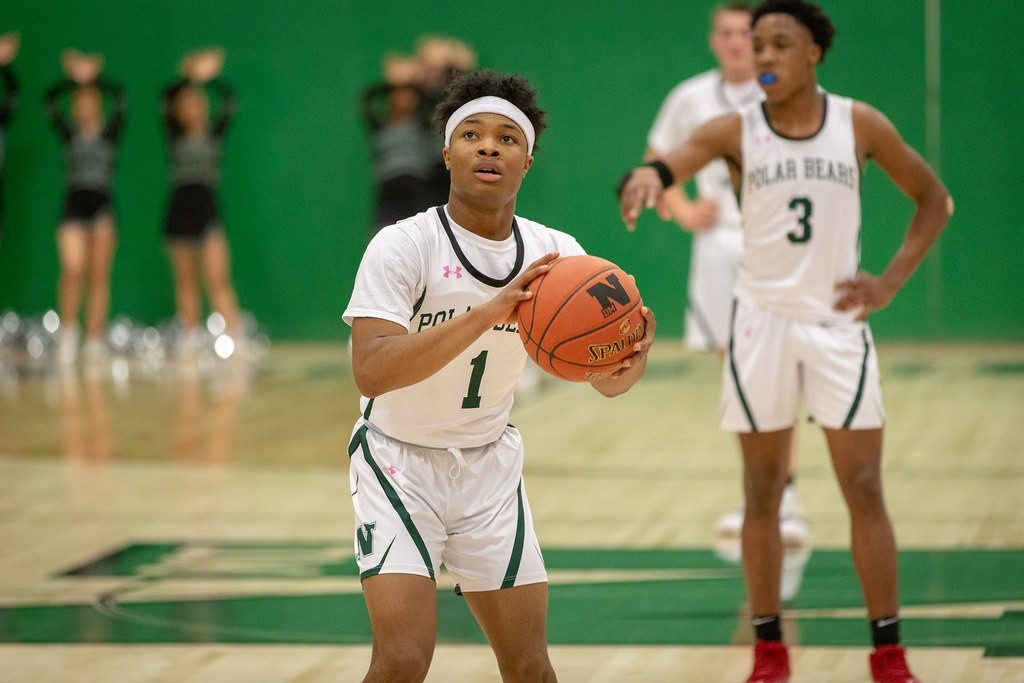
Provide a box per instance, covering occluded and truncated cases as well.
[362,41,475,226]
[343,72,656,683]
[622,0,954,683]
[646,0,812,545]
[162,51,242,355]
[44,51,125,355]
[0,33,23,132]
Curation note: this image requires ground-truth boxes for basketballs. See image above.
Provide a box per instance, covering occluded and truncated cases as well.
[517,254,646,383]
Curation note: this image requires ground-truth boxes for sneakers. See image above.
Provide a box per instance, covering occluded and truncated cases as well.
[870,646,921,683]
[745,642,790,683]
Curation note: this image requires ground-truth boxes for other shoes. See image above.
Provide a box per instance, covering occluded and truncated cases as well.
[779,518,807,544]
[718,513,744,532]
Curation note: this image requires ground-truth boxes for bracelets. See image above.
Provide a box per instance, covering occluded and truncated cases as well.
[650,159,674,189]
